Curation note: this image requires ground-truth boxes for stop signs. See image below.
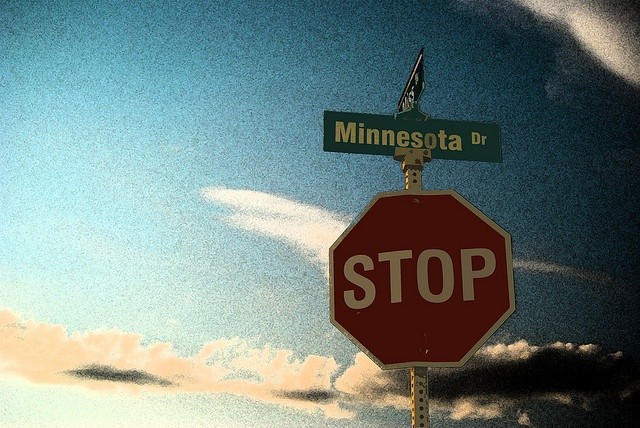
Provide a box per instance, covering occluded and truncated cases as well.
[329,191,517,368]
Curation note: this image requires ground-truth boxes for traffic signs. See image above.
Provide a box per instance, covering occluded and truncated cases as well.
[322,110,504,163]
